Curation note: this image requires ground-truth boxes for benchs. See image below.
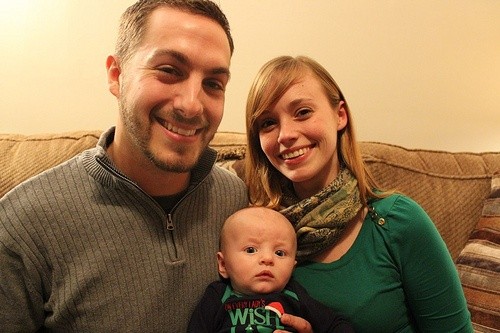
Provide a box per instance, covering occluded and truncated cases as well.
[0,130,500,333]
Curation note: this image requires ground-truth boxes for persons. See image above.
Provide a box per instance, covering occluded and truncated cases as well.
[187,207,332,333]
[0,0,310,333]
[225,55,475,333]
